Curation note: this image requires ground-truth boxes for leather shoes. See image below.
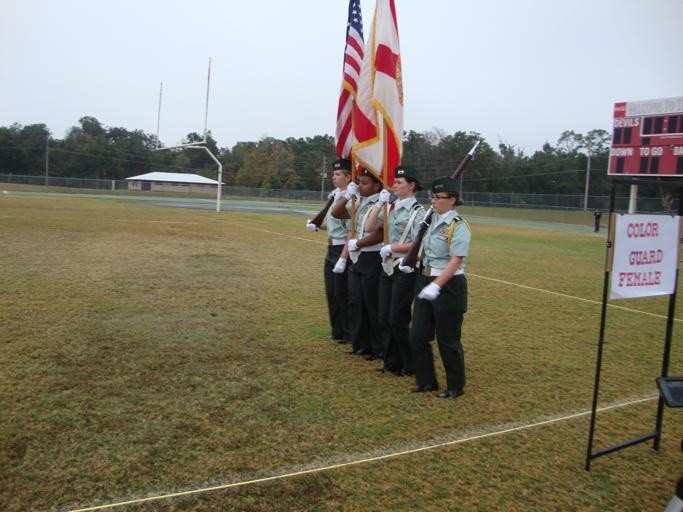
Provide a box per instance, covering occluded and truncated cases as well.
[325,335,413,377]
[436,387,464,399]
[410,382,439,394]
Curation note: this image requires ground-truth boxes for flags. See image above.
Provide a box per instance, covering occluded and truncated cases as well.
[350,1,406,195]
[333,1,365,177]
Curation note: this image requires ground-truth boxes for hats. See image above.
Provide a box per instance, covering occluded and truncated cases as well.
[361,166,380,182]
[393,165,425,192]
[331,157,352,171]
[430,176,461,194]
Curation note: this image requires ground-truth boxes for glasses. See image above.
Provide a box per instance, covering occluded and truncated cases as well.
[429,193,451,202]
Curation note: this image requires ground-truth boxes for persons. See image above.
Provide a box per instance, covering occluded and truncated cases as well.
[331,162,383,361]
[305,158,360,345]
[396,175,470,399]
[593,206,602,232]
[362,164,427,377]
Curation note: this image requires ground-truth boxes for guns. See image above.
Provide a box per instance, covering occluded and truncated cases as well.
[402,140,481,269]
[311,193,334,228]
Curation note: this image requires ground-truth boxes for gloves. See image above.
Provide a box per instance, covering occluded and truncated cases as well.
[398,257,416,275]
[347,238,359,253]
[379,244,392,260]
[344,181,360,201]
[378,188,391,207]
[417,281,442,302]
[331,257,347,274]
[306,218,317,230]
